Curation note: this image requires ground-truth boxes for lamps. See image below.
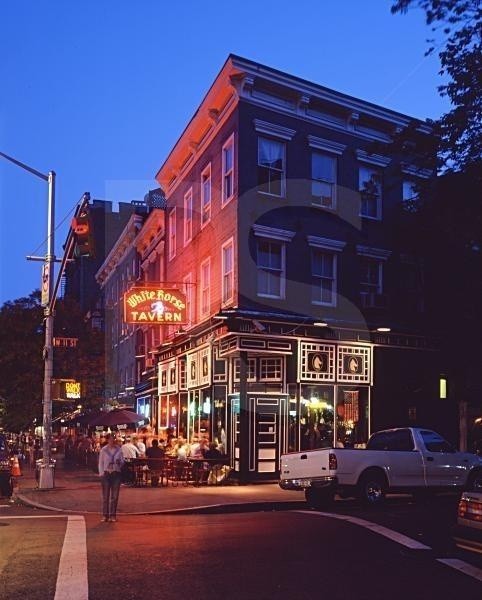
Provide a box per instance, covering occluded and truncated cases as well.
[368,327,392,334]
[313,322,329,328]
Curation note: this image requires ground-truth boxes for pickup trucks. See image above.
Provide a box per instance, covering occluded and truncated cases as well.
[278,426,481,505]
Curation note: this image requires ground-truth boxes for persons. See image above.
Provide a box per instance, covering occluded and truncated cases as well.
[97,434,126,523]
[121,436,221,489]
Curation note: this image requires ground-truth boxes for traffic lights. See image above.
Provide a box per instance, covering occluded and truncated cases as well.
[72,212,96,261]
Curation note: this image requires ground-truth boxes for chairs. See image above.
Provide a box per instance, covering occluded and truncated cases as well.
[72,446,230,490]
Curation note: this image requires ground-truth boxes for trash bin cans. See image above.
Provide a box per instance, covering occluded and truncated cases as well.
[38,464,54,490]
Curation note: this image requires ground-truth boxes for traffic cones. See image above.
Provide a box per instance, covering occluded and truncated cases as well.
[11,456,25,477]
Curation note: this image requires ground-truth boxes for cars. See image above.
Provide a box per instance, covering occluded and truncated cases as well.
[454,487,482,558]
[1,434,14,495]
